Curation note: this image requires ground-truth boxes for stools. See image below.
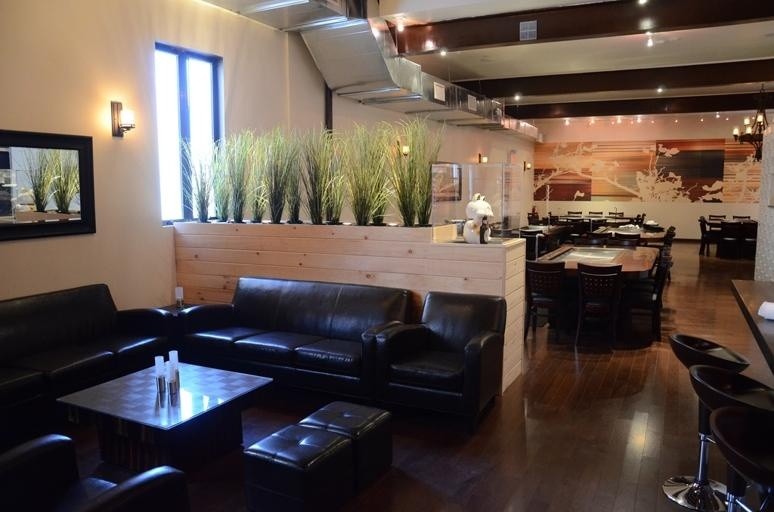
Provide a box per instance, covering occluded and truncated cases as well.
[708,405,774,512]
[240,424,354,511]
[298,399,394,478]
[688,363,774,512]
[661,333,752,512]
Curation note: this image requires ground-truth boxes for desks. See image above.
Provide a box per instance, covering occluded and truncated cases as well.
[728,279,774,376]
[157,302,200,314]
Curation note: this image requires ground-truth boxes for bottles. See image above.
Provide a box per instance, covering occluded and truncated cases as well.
[480,214,489,243]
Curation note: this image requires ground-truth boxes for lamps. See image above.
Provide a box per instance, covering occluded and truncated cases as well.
[523,160,531,171]
[478,153,488,164]
[397,142,409,158]
[109,99,136,138]
[731,83,771,164]
[174,286,185,307]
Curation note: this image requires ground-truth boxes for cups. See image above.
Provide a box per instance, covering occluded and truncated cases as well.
[176,296,185,308]
[156,369,181,396]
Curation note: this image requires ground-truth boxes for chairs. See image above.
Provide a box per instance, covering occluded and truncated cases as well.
[697,212,758,260]
[375,289,508,424]
[0,432,189,511]
[514,209,677,354]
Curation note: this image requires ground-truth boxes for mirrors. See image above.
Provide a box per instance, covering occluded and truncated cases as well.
[0,126,97,243]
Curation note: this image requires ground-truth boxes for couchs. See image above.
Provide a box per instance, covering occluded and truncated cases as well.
[1,280,174,420]
[177,274,413,398]
